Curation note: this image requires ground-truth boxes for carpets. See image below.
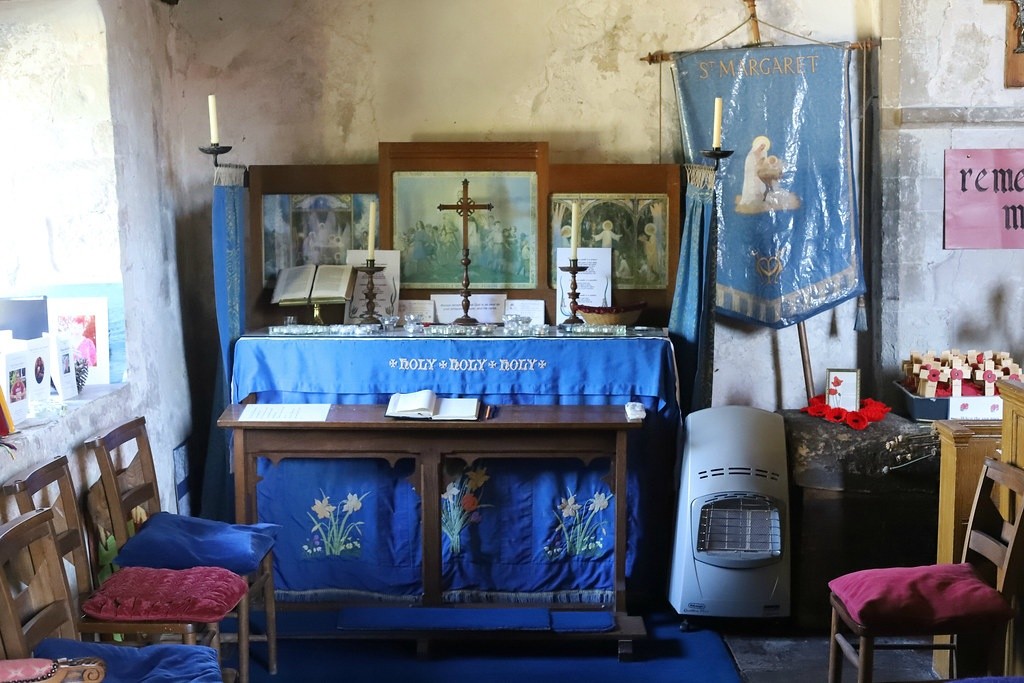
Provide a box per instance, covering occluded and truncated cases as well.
[216,592,745,683]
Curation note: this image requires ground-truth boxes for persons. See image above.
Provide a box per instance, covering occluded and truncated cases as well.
[400,209,531,276]
[63,316,96,375]
[10,375,26,402]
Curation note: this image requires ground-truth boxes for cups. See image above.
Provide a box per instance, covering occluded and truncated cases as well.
[268,316,627,336]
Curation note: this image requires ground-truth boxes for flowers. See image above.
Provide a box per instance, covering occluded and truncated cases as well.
[800,394,892,430]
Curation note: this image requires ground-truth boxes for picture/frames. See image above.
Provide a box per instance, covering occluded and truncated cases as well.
[826,369,861,411]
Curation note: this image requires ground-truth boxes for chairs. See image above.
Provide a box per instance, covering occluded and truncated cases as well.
[0,416,277,683]
[828,456,1024,683]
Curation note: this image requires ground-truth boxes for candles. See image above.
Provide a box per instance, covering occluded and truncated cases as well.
[368,203,376,259]
[208,94,219,143]
[572,203,579,260]
[712,97,722,147]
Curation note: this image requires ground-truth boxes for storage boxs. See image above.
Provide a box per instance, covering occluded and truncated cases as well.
[894,379,1003,422]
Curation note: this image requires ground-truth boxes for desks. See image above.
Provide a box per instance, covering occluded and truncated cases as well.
[217,328,680,663]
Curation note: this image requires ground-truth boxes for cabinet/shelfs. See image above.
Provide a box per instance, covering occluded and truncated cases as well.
[990,375,1024,678]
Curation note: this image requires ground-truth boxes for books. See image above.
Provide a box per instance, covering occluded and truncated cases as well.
[384,389,482,421]
